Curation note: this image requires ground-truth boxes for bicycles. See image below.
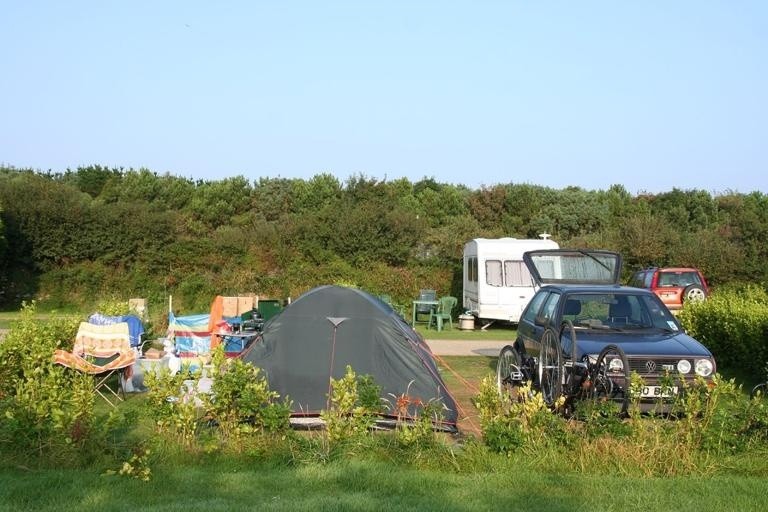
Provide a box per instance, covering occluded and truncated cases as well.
[496,318,631,420]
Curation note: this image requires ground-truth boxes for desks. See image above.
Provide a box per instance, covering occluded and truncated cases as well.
[412,301,441,328]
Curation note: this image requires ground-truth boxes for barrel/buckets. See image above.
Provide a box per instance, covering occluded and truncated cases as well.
[459,316,474,331]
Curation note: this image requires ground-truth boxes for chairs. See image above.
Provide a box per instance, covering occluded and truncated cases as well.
[52,322,136,410]
[415,290,458,331]
[563,300,632,324]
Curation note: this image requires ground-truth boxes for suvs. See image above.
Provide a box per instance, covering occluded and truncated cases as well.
[516,249,717,420]
[621,266,710,316]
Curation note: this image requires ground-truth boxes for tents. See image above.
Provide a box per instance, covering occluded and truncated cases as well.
[200,283,461,434]
